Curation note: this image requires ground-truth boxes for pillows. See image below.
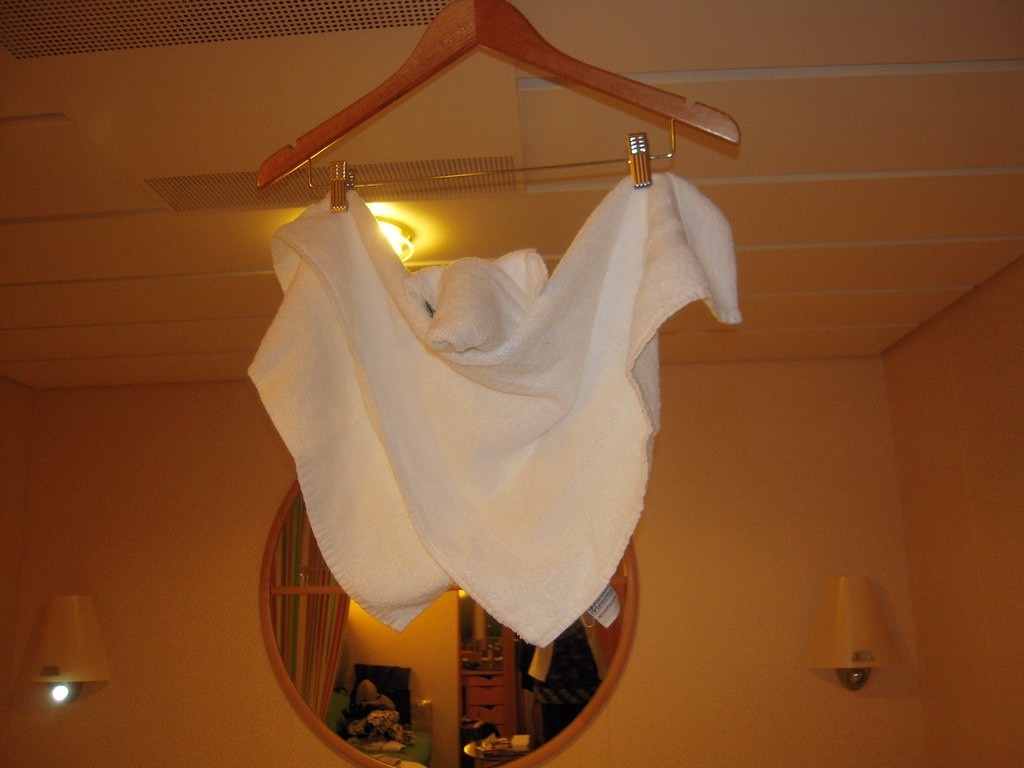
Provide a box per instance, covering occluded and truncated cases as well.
[351,663,413,726]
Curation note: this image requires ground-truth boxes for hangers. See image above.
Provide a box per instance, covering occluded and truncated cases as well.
[252,0,740,191]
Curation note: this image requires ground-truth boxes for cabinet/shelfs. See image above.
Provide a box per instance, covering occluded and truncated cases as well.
[458,589,518,740]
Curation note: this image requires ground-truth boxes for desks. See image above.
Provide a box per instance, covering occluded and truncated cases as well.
[464,736,546,768]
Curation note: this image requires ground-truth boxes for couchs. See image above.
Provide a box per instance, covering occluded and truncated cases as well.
[319,691,433,768]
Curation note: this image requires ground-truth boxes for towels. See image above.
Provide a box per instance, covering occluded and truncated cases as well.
[243,172,746,650]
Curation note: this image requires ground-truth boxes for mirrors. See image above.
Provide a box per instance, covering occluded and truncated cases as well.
[256,469,640,762]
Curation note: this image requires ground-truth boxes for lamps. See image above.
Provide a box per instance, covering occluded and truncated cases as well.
[811,570,895,692]
[23,589,113,707]
